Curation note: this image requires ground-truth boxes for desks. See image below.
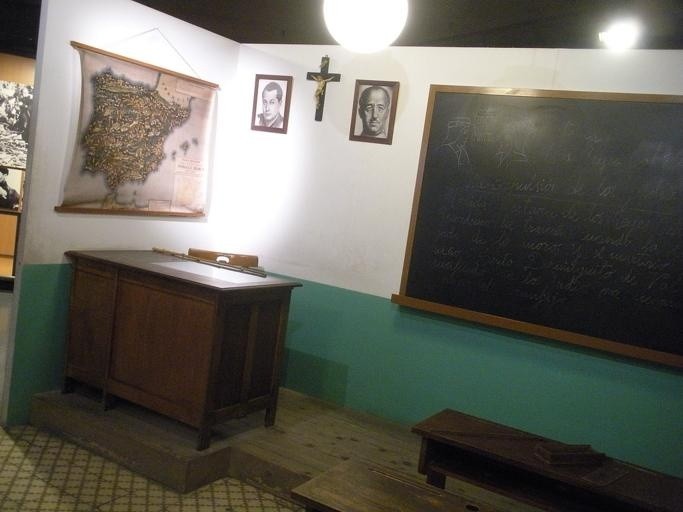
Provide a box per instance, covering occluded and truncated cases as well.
[412,406,682,511]
[289,459,498,512]
[64,246,303,453]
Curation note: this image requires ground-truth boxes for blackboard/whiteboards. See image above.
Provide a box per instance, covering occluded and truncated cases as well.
[400,84,681,368]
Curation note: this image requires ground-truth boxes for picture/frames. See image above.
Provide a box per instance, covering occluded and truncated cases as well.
[346,77,400,147]
[249,72,294,137]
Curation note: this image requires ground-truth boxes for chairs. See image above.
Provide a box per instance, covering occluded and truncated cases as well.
[188,248,260,268]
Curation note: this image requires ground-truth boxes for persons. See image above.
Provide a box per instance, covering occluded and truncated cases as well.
[257,82,284,128]
[0,166,21,209]
[358,86,391,139]
